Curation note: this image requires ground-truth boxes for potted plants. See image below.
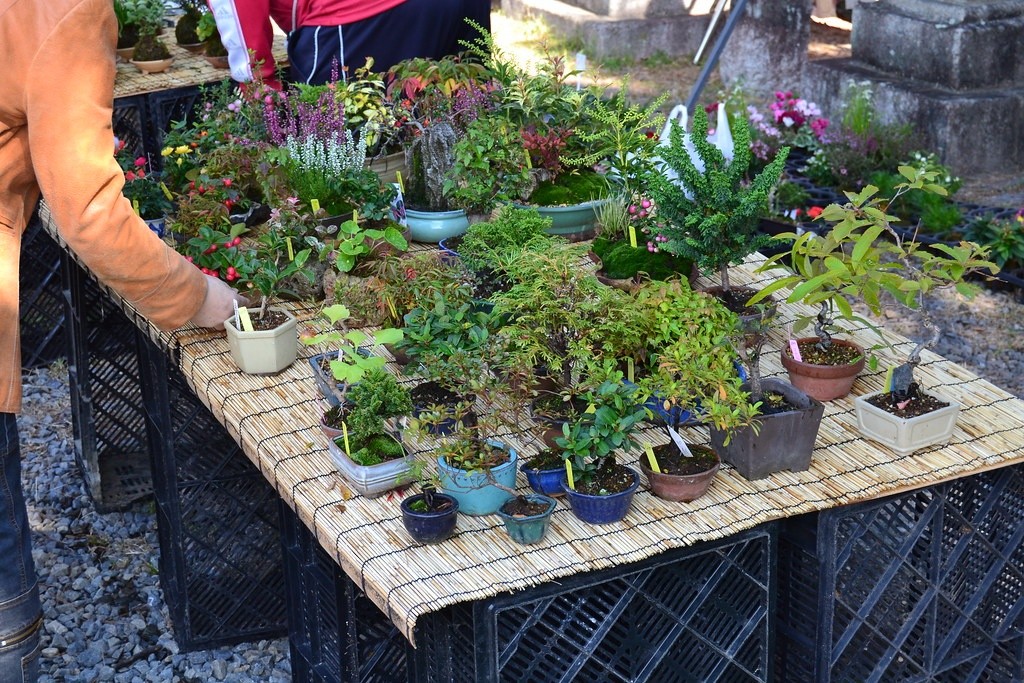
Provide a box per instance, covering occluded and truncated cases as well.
[111,0,1024,549]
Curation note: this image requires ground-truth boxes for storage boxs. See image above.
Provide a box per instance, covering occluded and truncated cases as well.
[135,331,286,653]
[19,77,246,375]
[274,461,1024,683]
[60,251,156,515]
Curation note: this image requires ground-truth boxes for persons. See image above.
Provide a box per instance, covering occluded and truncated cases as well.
[1,0,251,681]
[207,0,492,102]
